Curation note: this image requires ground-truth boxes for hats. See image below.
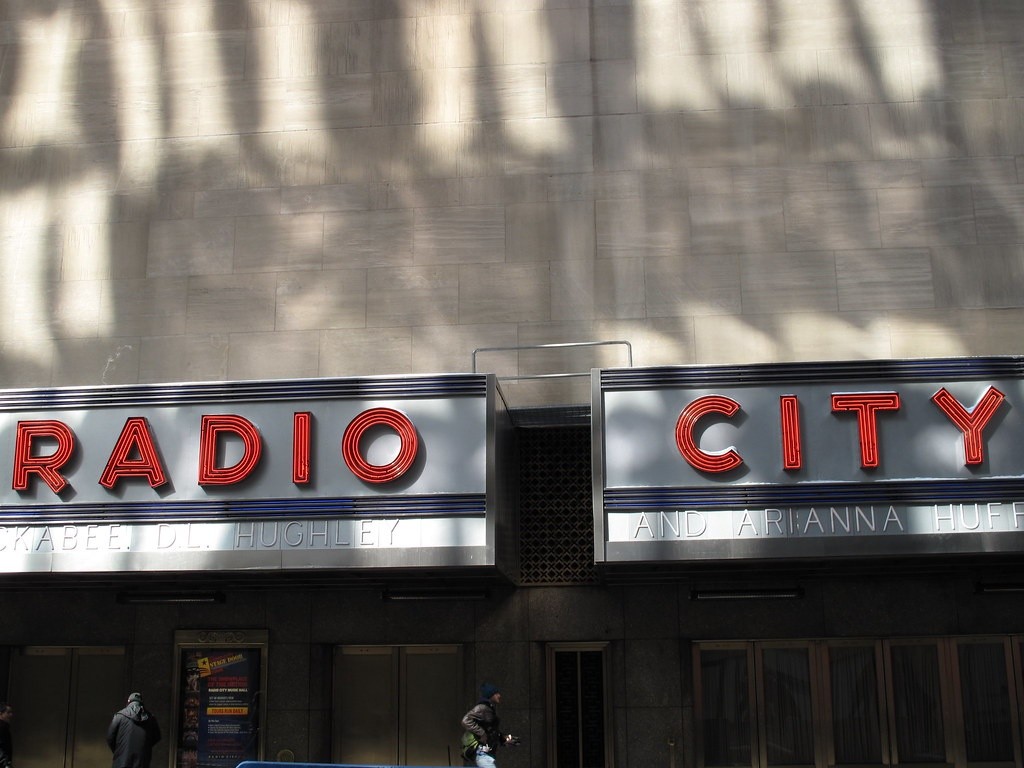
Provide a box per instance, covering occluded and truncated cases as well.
[127,692,143,702]
[480,682,499,699]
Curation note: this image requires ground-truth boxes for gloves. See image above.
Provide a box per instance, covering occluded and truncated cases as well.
[481,732,488,744]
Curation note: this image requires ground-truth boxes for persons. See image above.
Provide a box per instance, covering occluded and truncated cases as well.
[107,693,161,768]
[462,691,521,768]
[0,705,14,768]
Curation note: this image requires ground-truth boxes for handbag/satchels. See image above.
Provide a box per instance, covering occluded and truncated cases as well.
[460,730,480,762]
